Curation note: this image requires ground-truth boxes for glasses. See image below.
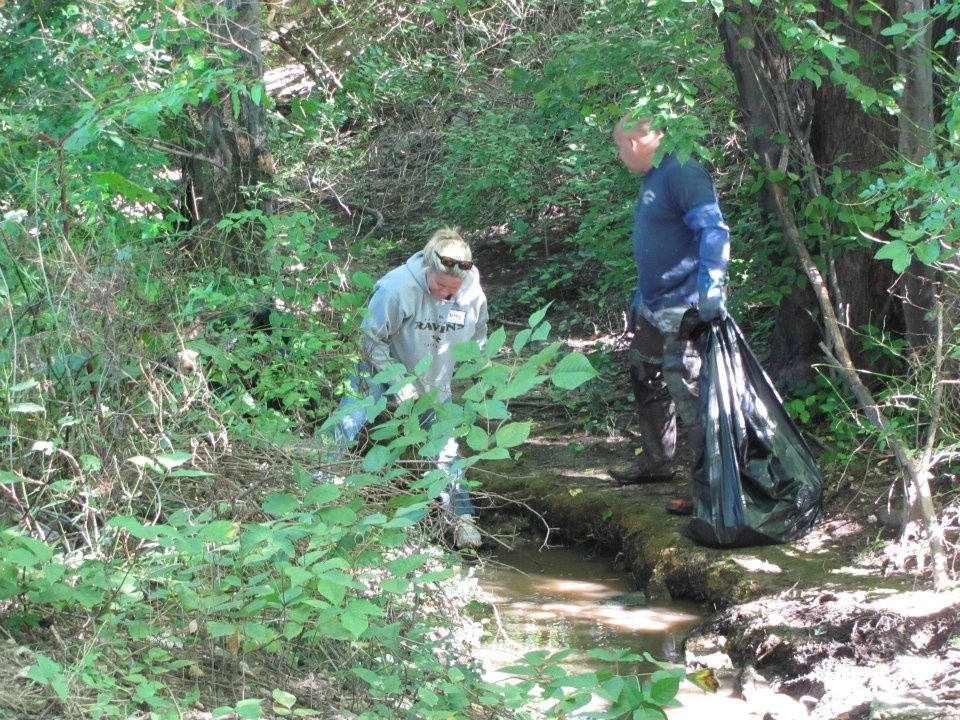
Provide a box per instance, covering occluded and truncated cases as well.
[434,251,474,271]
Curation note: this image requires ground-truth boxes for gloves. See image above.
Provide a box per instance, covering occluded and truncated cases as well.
[684,204,730,322]
[409,399,438,429]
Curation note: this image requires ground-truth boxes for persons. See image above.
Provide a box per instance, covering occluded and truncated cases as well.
[312,224,494,548]
[607,112,731,515]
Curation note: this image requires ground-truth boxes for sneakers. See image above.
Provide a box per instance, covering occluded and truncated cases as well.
[448,514,481,550]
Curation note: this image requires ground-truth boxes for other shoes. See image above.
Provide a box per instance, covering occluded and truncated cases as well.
[667,498,693,515]
[607,460,675,483]
